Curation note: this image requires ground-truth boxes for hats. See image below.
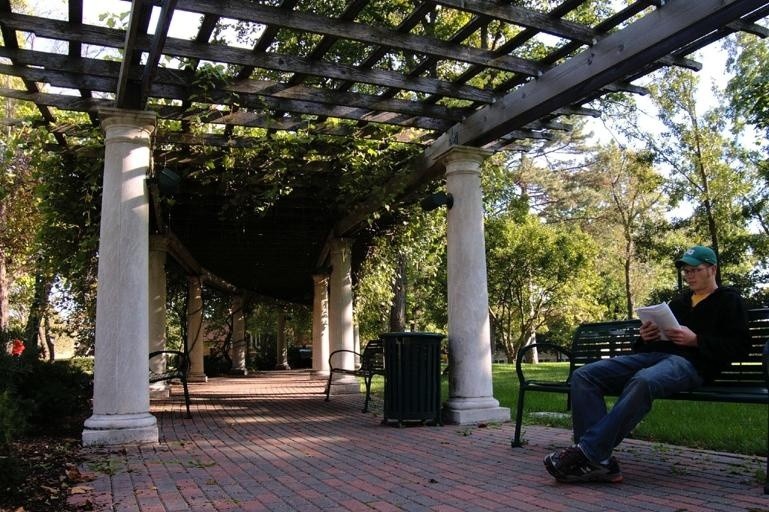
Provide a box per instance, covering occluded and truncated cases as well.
[675,246,717,268]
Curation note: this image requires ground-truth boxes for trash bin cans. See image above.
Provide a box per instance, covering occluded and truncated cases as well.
[379,332,447,428]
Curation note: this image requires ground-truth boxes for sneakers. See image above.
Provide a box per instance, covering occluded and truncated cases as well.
[543,444,622,483]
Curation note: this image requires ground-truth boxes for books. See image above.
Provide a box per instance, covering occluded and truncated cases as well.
[631,301,681,341]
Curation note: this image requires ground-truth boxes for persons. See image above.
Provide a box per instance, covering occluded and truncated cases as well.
[542,245,751,485]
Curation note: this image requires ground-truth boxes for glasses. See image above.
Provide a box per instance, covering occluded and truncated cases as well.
[684,264,712,273]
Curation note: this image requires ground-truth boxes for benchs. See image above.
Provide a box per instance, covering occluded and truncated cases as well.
[325,339,385,412]
[149,350,190,413]
[515,307,768,445]
